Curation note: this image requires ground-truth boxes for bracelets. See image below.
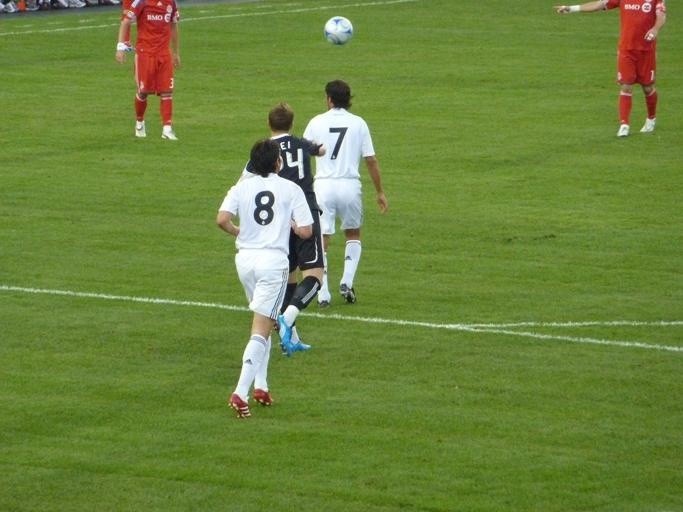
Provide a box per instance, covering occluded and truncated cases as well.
[570,5,581,15]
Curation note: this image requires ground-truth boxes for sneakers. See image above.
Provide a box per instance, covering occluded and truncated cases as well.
[640,117,656,133]
[290,340,311,352]
[339,278,356,304]
[161,125,179,141]
[228,392,252,418]
[273,315,292,357]
[316,292,331,306]
[135,120,146,138]
[617,124,630,137]
[252,388,273,406]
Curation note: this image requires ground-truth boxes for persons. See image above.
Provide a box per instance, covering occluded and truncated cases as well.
[116,1,184,142]
[306,79,390,308]
[553,2,667,139]
[1,1,122,15]
[237,102,327,360]
[215,137,316,419]
[121,1,133,56]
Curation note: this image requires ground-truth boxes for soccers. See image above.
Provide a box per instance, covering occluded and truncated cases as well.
[324,16,353,44]
